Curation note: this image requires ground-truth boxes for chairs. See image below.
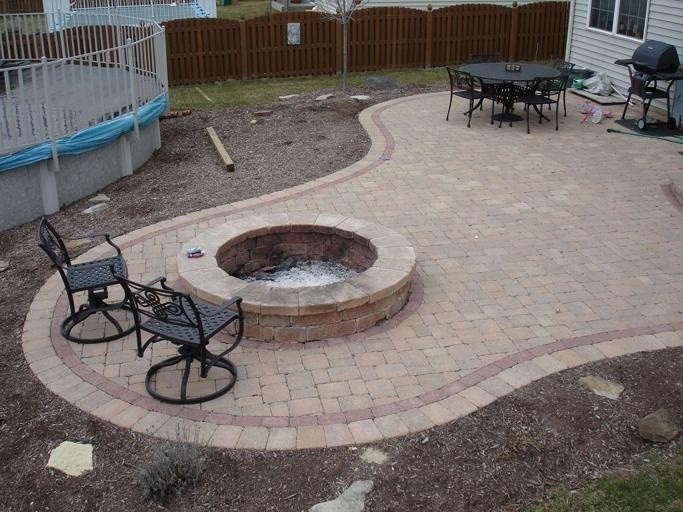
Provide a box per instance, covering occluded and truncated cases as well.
[442,64,569,136]
